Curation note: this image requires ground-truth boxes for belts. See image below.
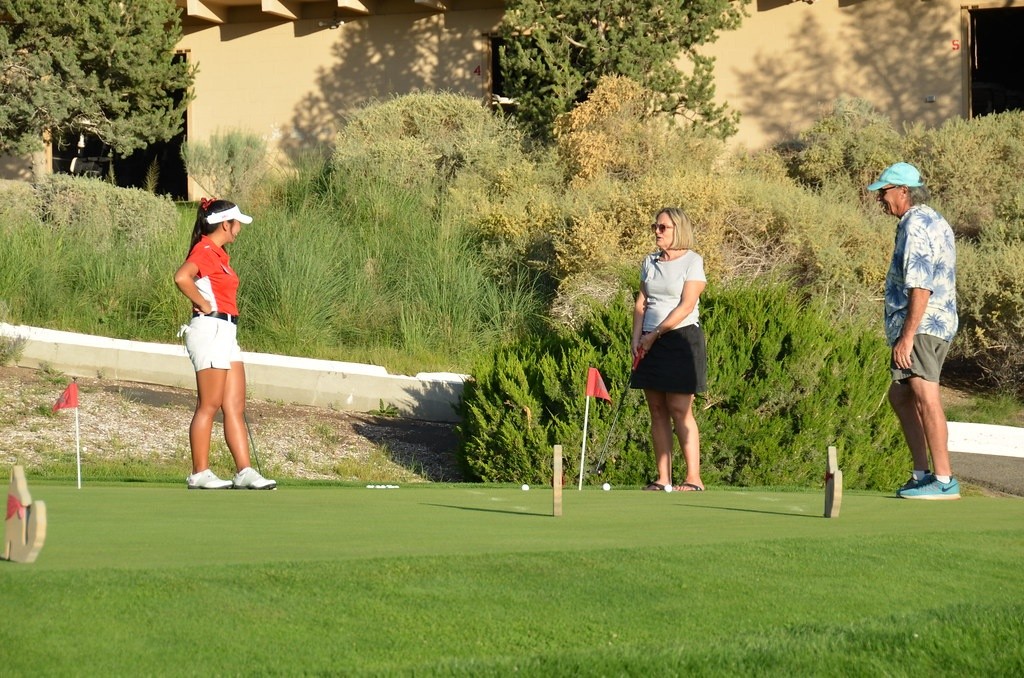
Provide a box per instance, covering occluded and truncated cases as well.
[191,310,238,325]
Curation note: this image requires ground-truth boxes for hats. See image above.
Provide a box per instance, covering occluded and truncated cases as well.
[206,203,254,226]
[866,162,925,192]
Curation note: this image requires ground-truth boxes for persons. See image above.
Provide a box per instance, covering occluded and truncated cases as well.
[632,207,707,494]
[174,197,276,490]
[868,161,961,500]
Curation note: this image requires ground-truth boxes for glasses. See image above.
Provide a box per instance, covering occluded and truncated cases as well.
[879,185,898,194]
[650,223,674,233]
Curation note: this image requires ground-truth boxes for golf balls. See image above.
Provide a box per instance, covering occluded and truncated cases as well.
[664,484,673,493]
[522,484,529,490]
[602,483,610,490]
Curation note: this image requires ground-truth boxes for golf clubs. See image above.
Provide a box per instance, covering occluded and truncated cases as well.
[244,411,263,476]
[592,330,649,477]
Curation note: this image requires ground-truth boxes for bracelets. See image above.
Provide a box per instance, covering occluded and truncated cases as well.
[654,327,661,338]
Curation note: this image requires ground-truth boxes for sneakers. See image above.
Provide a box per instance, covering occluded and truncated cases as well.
[899,475,961,499]
[895,475,932,498]
[232,467,278,490]
[188,470,233,490]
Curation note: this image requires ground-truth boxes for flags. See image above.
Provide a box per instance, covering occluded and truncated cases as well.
[586,367,612,408]
[51,381,78,415]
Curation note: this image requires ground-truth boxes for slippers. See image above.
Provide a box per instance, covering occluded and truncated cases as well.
[671,483,706,492]
[644,481,666,492]
